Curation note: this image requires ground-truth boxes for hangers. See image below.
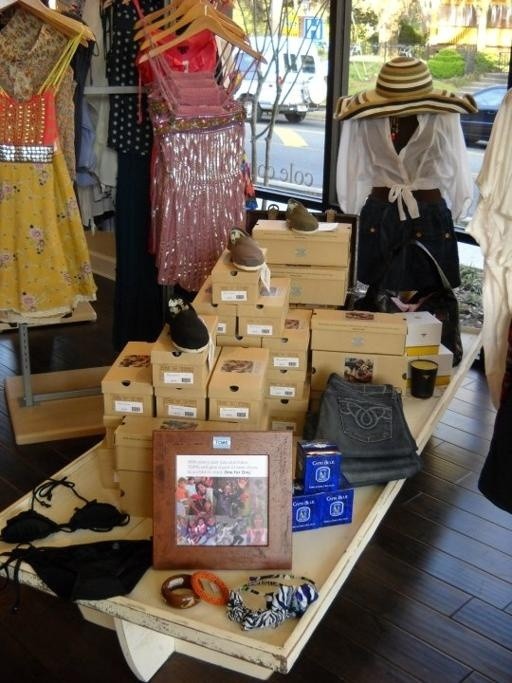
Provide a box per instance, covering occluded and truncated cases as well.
[0,0,270,77]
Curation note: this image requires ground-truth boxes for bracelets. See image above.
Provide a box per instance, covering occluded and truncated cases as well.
[161,571,230,609]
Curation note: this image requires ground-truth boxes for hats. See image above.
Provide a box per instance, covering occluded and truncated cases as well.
[332,56,479,121]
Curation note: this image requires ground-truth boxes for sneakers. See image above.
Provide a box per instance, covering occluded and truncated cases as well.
[286,197,319,234]
[163,294,212,354]
[227,227,266,271]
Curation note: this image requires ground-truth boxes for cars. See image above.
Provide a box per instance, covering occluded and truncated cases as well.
[459,85,511,143]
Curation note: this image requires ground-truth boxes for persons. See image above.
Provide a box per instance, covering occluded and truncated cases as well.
[177,476,267,545]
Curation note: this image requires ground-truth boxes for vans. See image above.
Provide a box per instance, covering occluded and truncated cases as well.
[231,34,326,120]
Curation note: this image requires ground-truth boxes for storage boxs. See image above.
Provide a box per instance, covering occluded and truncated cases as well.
[191,219,454,437]
[101,316,272,501]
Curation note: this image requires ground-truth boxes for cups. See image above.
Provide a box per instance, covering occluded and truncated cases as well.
[408,359,437,400]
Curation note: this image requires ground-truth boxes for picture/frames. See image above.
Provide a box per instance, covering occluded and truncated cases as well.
[147,427,297,570]
